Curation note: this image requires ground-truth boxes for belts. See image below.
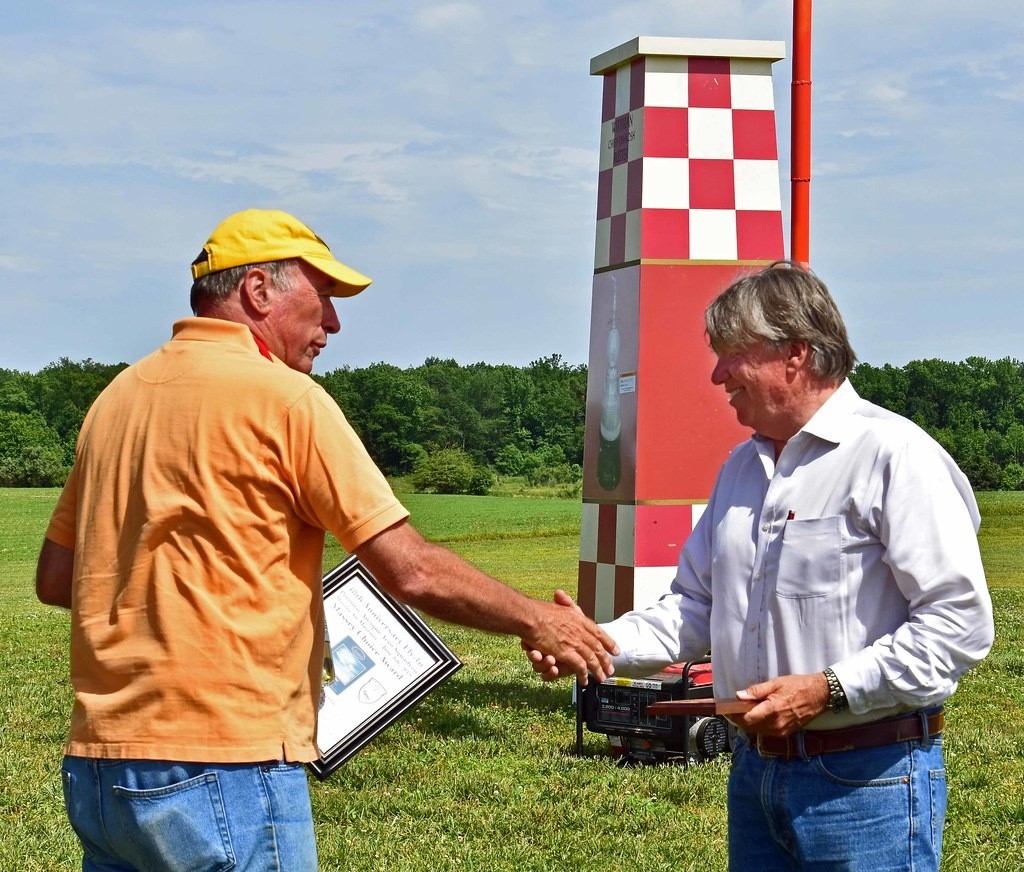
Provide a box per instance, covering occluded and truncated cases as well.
[746,707,944,758]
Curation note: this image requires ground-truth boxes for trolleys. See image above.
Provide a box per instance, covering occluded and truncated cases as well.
[574,655,739,767]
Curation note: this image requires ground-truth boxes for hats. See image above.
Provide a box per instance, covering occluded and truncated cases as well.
[190,208,373,297]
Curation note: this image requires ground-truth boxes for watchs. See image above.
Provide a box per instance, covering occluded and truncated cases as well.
[823,668,848,714]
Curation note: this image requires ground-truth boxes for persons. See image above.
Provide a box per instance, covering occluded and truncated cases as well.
[522,260,994,872]
[36,208,621,871]
